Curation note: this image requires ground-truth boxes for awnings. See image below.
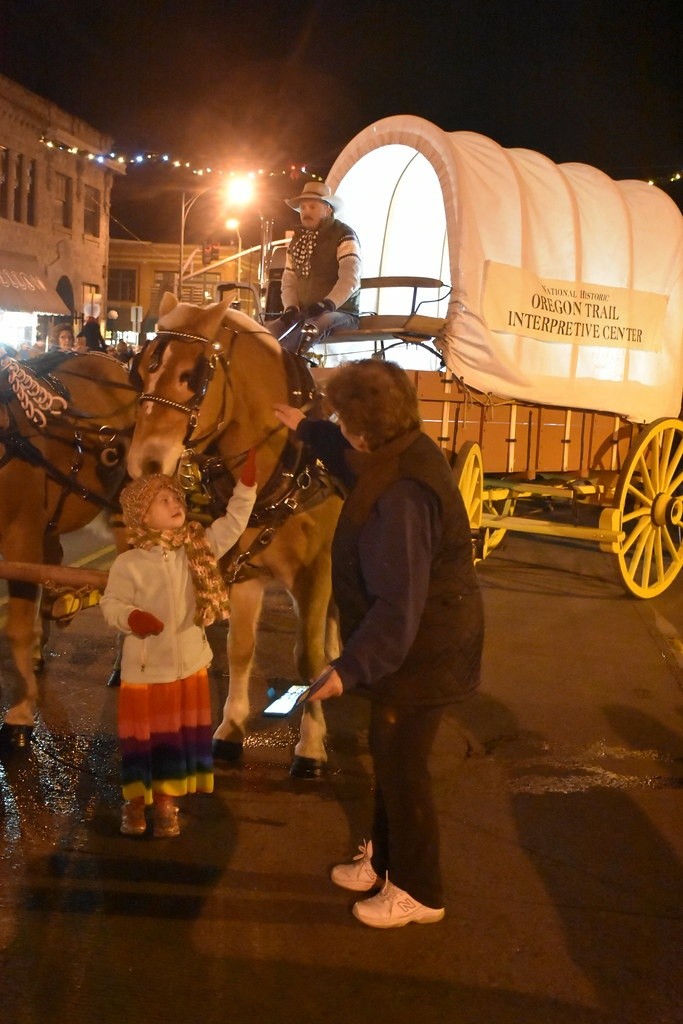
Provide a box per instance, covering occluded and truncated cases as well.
[0,249,72,317]
[141,315,159,334]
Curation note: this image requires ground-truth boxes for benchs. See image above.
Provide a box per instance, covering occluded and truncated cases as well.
[317,275,452,371]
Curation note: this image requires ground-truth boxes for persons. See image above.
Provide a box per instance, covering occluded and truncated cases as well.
[16,322,138,371]
[263,181,361,356]
[268,357,486,929]
[98,446,259,839]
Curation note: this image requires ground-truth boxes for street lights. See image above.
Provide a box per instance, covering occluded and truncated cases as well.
[174,168,257,311]
[107,310,119,346]
[228,216,244,308]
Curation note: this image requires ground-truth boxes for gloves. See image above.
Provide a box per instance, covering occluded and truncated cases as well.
[308,301,333,317]
[280,307,296,325]
[128,609,164,639]
[240,447,258,487]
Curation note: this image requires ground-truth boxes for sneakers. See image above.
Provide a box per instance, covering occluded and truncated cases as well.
[353,882,445,928]
[331,854,388,892]
[149,804,180,837]
[119,797,147,835]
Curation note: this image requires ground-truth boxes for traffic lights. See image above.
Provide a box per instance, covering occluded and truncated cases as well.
[209,242,220,261]
[202,241,211,265]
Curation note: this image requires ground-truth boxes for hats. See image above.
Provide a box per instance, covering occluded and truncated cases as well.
[120,472,186,530]
[284,181,344,213]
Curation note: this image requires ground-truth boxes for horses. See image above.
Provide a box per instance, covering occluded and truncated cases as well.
[0,347,138,750]
[129,289,367,777]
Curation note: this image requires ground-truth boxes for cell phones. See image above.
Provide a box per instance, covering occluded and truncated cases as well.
[262,683,310,720]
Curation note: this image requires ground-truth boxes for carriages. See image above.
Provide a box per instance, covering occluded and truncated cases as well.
[0,120,683,795]
